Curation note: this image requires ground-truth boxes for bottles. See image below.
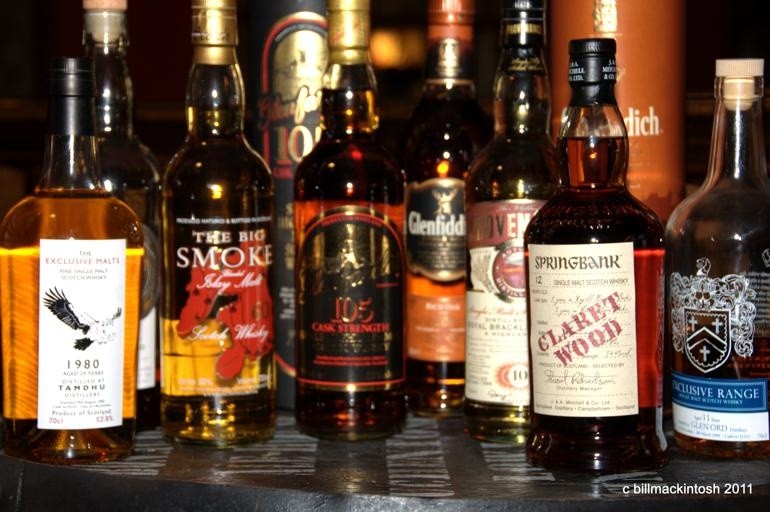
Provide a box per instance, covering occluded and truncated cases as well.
[1,54,145,466]
[463,0,564,446]
[399,0,492,415]
[550,0,688,224]
[238,0,329,411]
[80,0,163,431]
[663,57,770,460]
[293,0,408,439]
[155,0,279,446]
[523,38,668,475]
